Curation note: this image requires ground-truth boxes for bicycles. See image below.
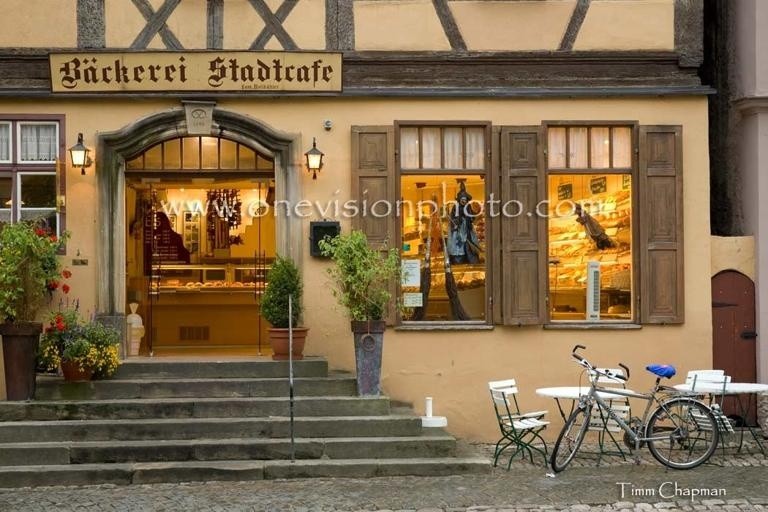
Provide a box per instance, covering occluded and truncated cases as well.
[550,344,720,473]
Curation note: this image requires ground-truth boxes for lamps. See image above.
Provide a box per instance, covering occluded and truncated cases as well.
[303,136,325,180]
[66,131,94,178]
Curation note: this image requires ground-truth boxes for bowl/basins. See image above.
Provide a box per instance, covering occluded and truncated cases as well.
[421,415,448,428]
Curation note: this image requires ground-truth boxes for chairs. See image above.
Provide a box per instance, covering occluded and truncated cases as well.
[487,365,768,472]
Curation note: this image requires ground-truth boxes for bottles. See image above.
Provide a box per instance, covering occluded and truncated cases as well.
[424,396,434,417]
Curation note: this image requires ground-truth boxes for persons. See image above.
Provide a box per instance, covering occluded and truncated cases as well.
[447,190,481,267]
[574,203,611,250]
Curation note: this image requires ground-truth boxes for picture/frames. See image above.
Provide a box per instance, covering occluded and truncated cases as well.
[166,213,178,235]
[182,210,202,264]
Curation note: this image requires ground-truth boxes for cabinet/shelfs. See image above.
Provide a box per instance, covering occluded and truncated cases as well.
[400,258,487,323]
[149,259,275,347]
[548,196,631,314]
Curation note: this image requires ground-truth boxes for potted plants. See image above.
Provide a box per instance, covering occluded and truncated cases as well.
[0,223,53,401]
[256,244,311,361]
[58,338,96,383]
[319,225,419,399]
